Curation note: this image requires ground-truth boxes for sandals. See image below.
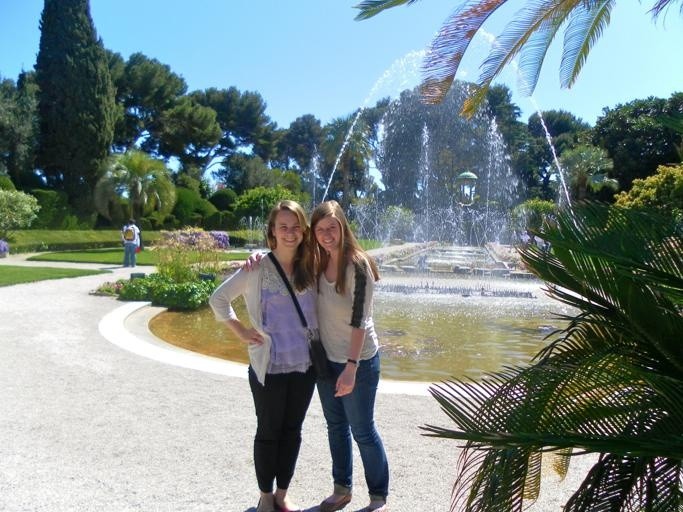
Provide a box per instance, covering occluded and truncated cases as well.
[256,494,274,512]
[273,494,302,512]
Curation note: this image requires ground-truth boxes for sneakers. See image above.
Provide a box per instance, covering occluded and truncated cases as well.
[368,500,387,512]
[321,493,351,512]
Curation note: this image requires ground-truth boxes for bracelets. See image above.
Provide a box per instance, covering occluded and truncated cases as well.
[347,359,360,367]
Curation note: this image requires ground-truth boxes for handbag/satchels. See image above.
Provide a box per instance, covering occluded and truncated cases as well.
[306,337,332,379]
[121,228,134,241]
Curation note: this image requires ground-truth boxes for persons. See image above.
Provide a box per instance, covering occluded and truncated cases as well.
[209,199,321,507]
[120,218,141,269]
[240,198,392,511]
